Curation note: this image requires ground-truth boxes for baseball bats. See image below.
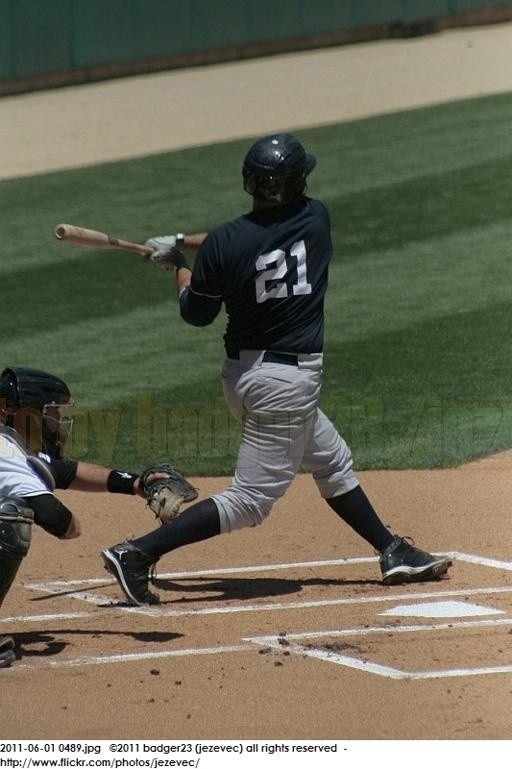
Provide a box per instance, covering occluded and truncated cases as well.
[56,225,155,255]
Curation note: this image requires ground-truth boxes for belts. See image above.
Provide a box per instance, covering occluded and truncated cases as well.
[226,349,297,367]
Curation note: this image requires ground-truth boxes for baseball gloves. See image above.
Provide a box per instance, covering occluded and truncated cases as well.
[139,462,197,523]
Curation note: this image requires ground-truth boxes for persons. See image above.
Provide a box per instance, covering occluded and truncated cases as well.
[98,131,453,609]
[1,365,169,670]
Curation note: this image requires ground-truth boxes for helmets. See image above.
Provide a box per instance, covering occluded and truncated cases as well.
[241,134,317,204]
[0,367,75,462]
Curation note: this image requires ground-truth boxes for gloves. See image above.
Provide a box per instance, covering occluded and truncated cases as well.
[139,234,191,280]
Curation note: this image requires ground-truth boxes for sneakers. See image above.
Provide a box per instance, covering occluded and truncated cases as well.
[379,534,453,586]
[101,539,160,607]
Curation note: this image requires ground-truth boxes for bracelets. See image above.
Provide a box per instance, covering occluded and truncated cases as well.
[106,469,139,495]
